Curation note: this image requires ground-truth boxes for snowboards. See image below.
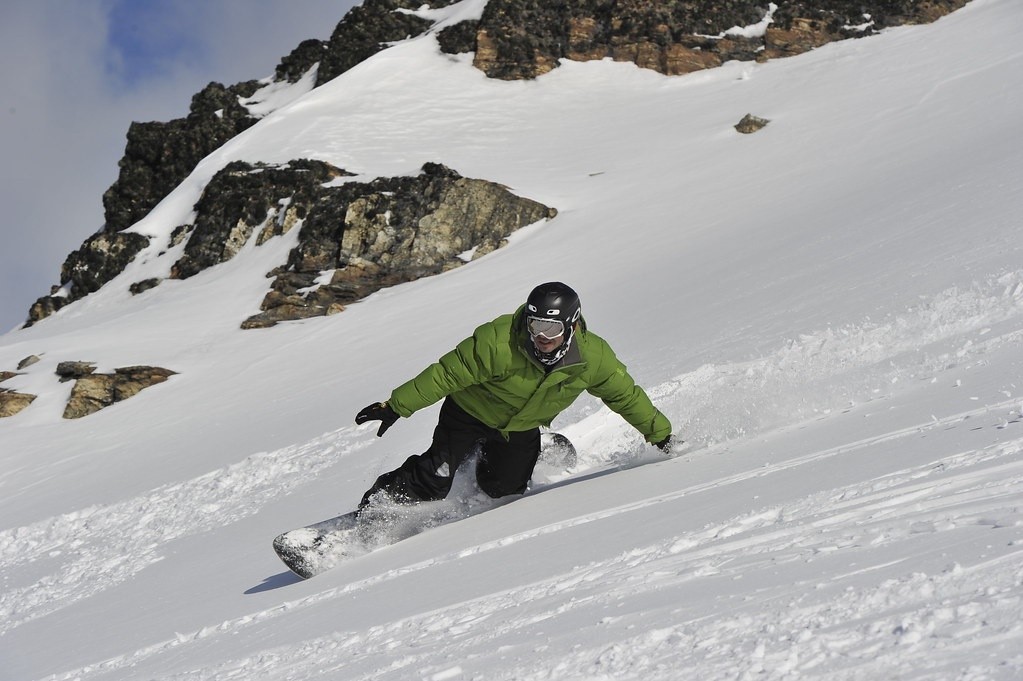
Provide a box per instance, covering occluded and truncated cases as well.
[271,430,580,579]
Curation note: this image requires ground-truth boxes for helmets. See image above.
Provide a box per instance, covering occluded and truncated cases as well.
[525,281,581,332]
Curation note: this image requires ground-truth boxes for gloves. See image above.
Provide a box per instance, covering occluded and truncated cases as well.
[656,433,685,457]
[355,400,401,437]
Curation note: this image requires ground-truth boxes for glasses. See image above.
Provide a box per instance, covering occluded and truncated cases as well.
[527,316,564,339]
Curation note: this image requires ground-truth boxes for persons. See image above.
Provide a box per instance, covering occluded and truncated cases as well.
[354,281,683,509]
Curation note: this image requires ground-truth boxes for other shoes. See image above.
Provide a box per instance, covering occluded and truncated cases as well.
[357,480,397,522]
